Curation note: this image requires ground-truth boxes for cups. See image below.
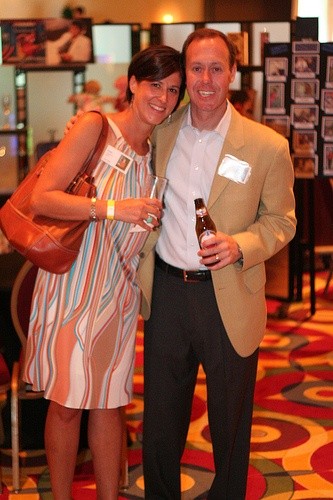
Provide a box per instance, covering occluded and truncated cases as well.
[140,174,169,226]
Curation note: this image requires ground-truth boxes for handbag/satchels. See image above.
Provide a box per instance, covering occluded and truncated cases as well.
[0,111,108,275]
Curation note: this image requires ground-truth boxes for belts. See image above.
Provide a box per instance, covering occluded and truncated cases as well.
[152,254,212,282]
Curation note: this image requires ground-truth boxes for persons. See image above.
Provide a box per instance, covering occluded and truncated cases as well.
[22,45,185,500]
[64,29,296,500]
[119,158,126,168]
[269,57,333,174]
[2,6,92,64]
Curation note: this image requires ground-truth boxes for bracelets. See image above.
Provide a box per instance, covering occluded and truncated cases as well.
[107,199,114,220]
[90,197,96,222]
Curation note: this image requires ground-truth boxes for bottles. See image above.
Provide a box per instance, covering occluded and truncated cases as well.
[194,198,220,266]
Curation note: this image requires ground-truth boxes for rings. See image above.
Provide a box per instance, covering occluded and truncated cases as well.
[215,254,219,260]
[148,217,152,222]
